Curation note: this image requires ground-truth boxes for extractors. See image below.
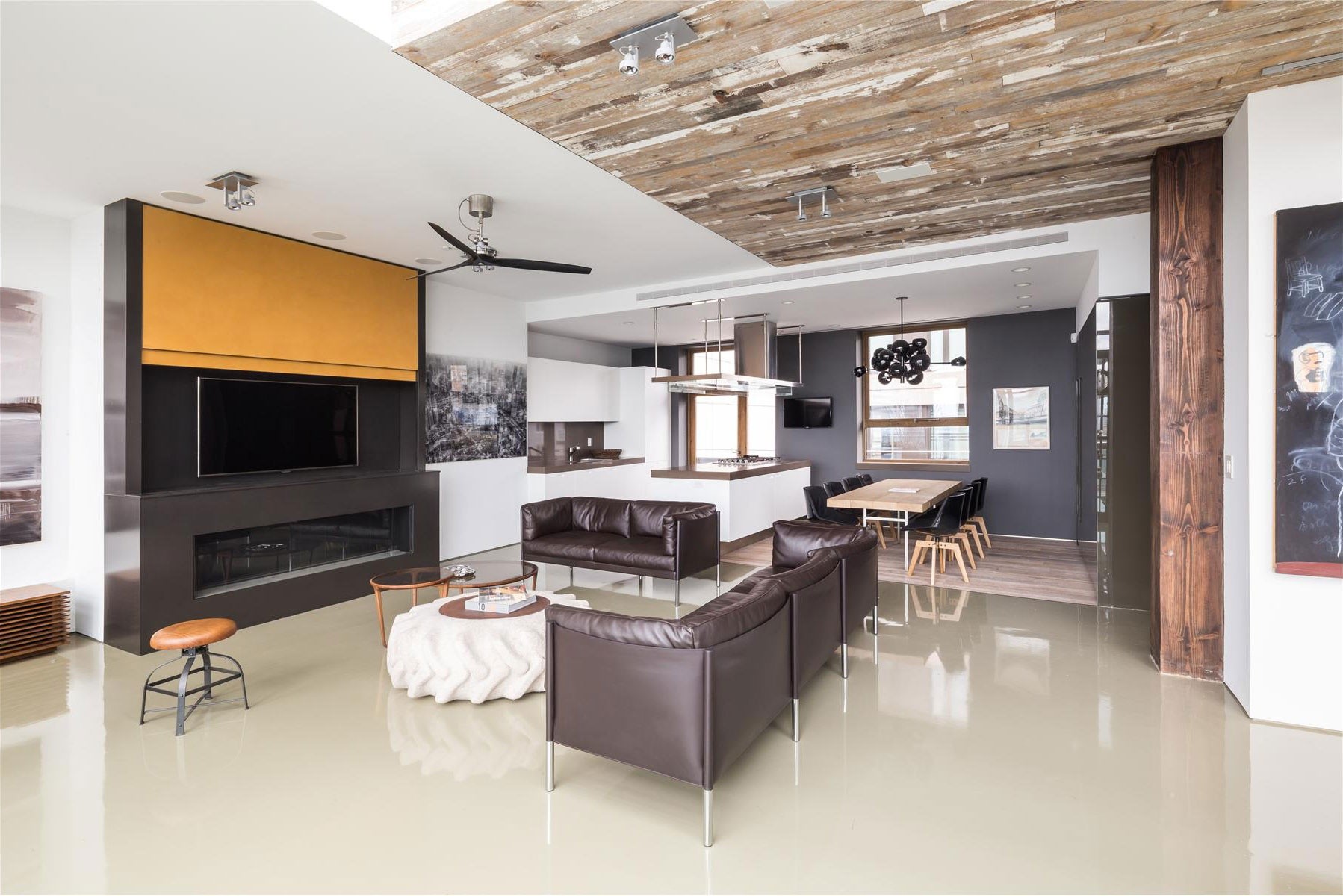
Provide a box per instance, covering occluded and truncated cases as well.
[735,321,778,390]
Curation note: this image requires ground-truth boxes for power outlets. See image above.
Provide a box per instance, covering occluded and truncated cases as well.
[588,437,592,446]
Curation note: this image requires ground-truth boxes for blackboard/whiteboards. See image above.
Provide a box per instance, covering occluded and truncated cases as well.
[1276,203,1342,577]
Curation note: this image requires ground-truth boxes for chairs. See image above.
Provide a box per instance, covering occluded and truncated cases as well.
[948,479,984,562]
[804,485,863,528]
[970,476,992,549]
[856,471,897,542]
[918,486,975,570]
[898,491,970,584]
[842,474,876,511]
[824,480,886,549]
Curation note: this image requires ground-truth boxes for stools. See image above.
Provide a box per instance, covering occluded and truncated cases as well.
[138,618,249,735]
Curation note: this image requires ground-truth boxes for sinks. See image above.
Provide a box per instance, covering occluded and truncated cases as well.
[564,458,614,465]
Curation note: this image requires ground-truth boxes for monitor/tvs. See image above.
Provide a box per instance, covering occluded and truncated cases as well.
[198,377,359,477]
[783,397,832,428]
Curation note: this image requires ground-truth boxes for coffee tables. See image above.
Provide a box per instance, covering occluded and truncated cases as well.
[369,566,454,648]
[388,586,593,698]
[431,559,539,596]
[214,537,316,583]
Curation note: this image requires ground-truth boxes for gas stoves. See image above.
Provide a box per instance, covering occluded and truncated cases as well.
[713,455,782,464]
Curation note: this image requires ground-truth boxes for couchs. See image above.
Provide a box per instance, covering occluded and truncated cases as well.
[517,496,721,607]
[541,520,880,845]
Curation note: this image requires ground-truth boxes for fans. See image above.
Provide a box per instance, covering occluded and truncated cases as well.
[405,193,592,282]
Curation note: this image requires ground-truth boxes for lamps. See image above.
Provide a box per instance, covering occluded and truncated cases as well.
[786,185,838,221]
[849,294,966,387]
[202,170,260,213]
[609,16,699,76]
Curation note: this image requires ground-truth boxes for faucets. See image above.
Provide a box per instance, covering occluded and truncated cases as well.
[569,446,580,462]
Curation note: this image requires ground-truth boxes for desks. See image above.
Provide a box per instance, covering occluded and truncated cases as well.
[829,476,963,573]
